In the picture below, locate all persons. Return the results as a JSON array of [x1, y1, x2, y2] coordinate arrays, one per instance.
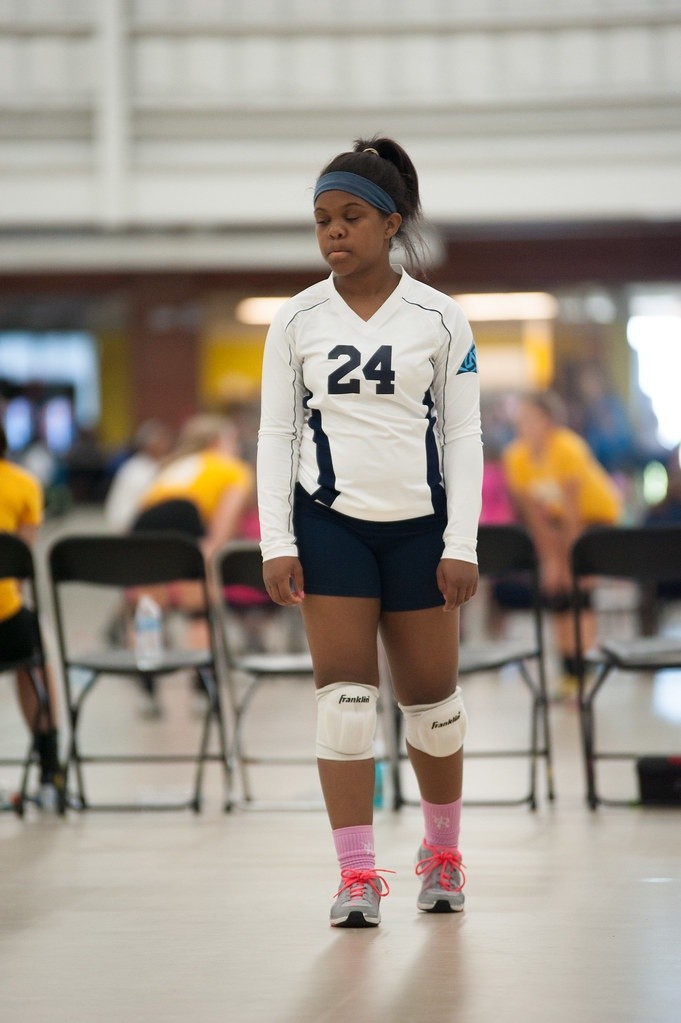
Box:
[[104, 350, 680, 718], [252, 135, 486, 924], [0, 422, 68, 790]]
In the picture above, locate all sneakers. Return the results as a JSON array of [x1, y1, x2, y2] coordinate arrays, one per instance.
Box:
[[330, 876, 383, 927], [418, 838, 466, 912]]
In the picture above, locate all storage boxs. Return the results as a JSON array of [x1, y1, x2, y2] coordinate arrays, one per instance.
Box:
[[636, 756, 681, 810]]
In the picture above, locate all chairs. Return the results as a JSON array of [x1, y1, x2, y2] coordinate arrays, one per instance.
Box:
[[388, 524, 558, 810], [48, 533, 237, 812], [217, 543, 319, 803], [562, 526, 681, 809], [0, 533, 71, 816]]
